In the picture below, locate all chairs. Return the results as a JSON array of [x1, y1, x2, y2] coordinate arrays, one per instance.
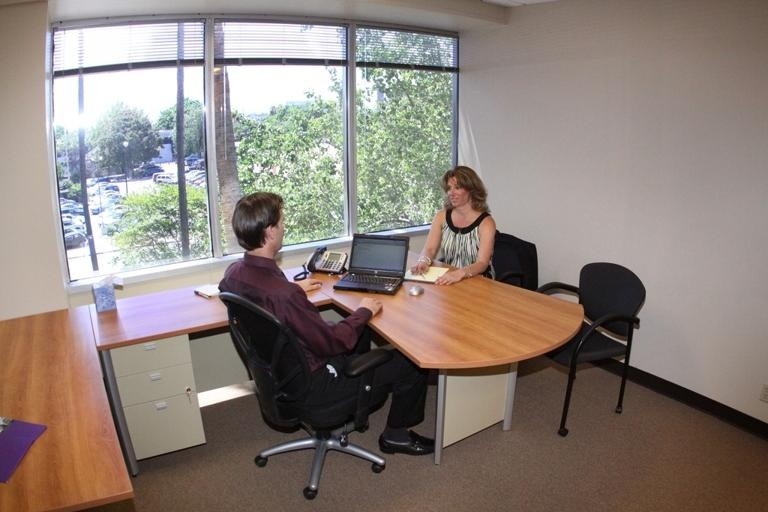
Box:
[[438, 226, 539, 287], [218, 293, 386, 501], [537, 261, 647, 437]]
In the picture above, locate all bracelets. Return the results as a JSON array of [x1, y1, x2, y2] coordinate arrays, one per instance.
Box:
[[417, 255, 432, 266], [463, 266, 472, 279]]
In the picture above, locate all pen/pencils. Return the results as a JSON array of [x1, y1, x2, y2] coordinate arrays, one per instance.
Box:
[[194, 290, 210, 299]]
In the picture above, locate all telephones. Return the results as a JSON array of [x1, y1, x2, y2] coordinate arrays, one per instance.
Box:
[[303, 246, 348, 274]]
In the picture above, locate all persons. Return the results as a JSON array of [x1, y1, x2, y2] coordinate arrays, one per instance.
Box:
[[218, 192, 437, 456], [411, 166, 497, 286]]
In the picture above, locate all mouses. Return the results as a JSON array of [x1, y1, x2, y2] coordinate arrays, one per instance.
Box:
[[408, 284, 424, 295]]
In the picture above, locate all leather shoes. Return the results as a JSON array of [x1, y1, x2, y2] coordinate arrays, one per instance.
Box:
[[379, 430, 434, 455]]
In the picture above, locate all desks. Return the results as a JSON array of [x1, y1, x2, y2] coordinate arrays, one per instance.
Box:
[[89, 272, 333, 476], [0, 305, 135, 512], [307, 247, 586, 465]]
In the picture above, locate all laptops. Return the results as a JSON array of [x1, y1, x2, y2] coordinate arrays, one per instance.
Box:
[[332, 232, 408, 298]]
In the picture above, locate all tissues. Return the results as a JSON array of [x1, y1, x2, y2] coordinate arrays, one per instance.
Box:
[[91, 273, 118, 314]]
